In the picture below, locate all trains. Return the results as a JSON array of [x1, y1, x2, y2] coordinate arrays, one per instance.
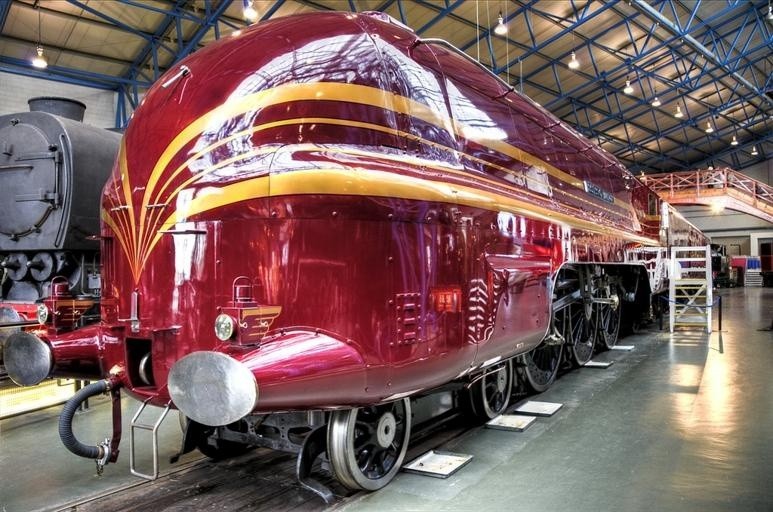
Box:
[[0, 90, 125, 353], [0, 2, 727, 510]]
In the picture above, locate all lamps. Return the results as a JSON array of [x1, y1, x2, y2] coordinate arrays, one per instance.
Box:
[[566, 14, 581, 70], [493, 0, 508, 36], [31, 0, 48, 68], [622, 67, 760, 157]]
